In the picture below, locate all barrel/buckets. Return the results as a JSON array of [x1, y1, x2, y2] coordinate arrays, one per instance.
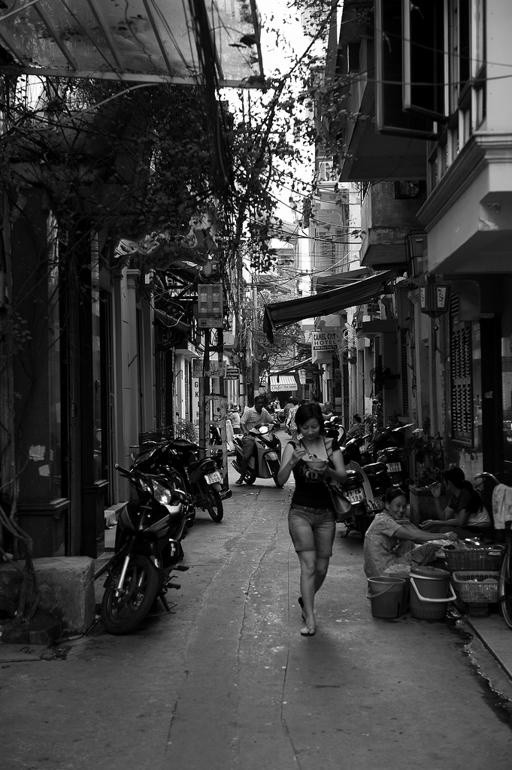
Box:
[[365, 574, 407, 619], [409, 566, 456, 619]]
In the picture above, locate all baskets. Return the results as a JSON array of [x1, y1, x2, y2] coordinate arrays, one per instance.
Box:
[[452, 571, 500, 602], [441, 545, 505, 570]]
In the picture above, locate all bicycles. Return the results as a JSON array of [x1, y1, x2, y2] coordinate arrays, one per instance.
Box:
[[474, 473, 511, 629]]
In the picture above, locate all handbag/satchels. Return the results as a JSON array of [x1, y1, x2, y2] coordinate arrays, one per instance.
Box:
[[323, 477, 351, 515]]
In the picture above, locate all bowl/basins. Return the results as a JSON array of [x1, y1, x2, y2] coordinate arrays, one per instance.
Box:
[[305, 460, 328, 470]]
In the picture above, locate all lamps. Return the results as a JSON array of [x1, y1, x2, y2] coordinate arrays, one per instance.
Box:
[[350, 335, 357, 365], [420, 270, 451, 359]]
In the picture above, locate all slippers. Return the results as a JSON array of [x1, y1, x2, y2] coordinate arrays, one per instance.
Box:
[[298, 597, 316, 636]]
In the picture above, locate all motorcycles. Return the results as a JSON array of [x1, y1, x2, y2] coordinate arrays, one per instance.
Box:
[[102, 437, 223, 634], [325, 415, 408, 537], [230, 420, 283, 488]]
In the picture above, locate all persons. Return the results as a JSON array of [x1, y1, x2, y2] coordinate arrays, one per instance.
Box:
[[419, 466, 491, 542], [277, 403, 346, 635], [226, 396, 365, 486], [364, 488, 458, 580]]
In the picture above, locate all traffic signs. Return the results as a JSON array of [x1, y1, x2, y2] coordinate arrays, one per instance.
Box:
[[221, 369, 240, 380], [312, 331, 340, 351]]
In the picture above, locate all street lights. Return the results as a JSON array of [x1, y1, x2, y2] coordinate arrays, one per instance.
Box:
[[419, 272, 449, 487], [200, 260, 220, 460]]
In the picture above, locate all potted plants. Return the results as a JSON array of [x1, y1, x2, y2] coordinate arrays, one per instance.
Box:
[[408, 454, 447, 529]]
